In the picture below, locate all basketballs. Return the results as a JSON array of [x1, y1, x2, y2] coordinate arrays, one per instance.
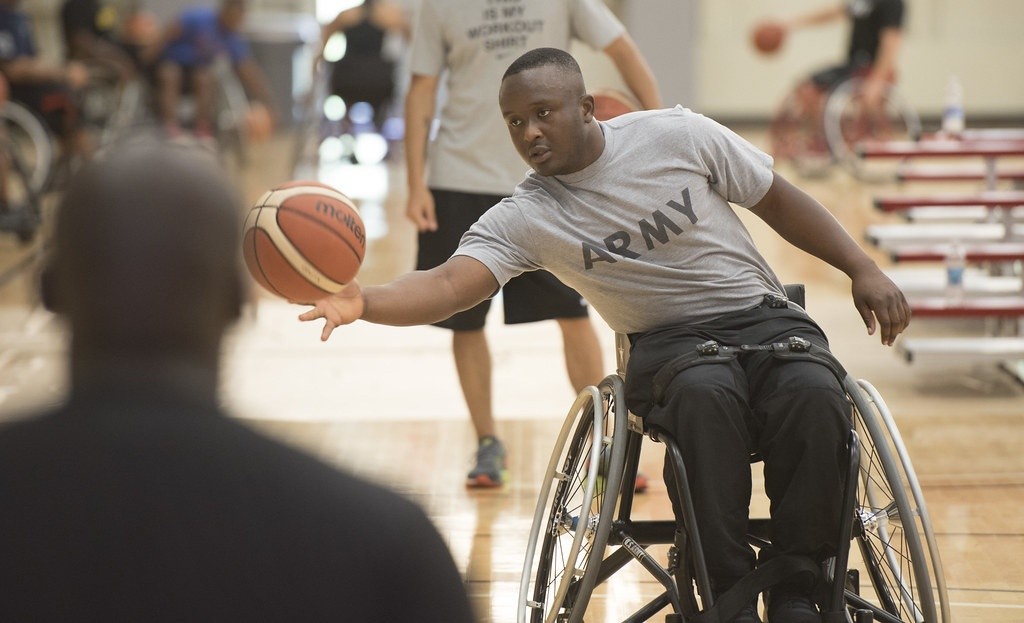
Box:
[[240, 179, 368, 303]]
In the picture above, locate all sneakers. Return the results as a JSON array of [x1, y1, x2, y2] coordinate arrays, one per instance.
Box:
[[465, 434, 509, 487], [585, 440, 648, 494]]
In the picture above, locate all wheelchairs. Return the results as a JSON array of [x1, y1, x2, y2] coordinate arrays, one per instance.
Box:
[[774, 66, 923, 181], [518, 284, 949, 623]]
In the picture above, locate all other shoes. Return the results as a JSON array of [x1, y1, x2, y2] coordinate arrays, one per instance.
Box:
[[768, 591, 822, 623], [725, 603, 762, 623]]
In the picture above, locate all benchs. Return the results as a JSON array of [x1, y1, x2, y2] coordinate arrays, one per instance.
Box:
[[858, 136, 1024, 386]]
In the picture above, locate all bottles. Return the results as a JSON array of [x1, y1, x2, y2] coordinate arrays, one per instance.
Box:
[[945, 244, 964, 299], [943, 82, 964, 137]]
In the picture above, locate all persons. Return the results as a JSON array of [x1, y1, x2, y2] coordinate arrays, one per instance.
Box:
[[0, 141, 475, 622], [287, 49, 910, 623], [312, 0, 405, 165], [406, 0, 665, 490], [0, 0, 269, 242], [775, 0, 905, 154]]
